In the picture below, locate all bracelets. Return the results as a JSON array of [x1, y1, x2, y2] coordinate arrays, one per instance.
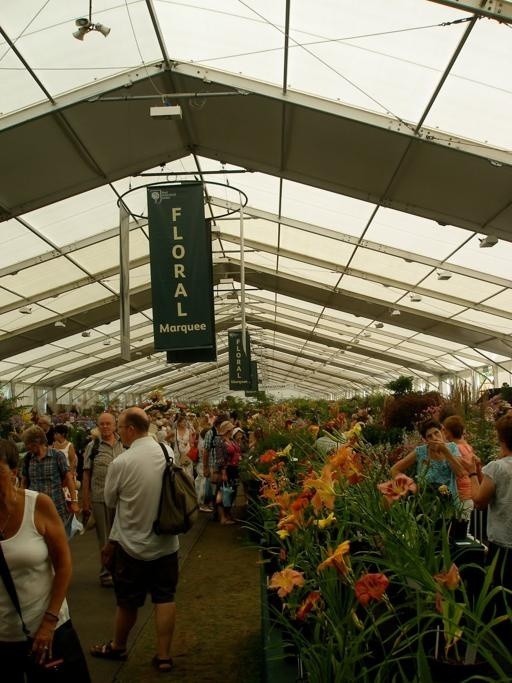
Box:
[[469, 472, 476, 477], [45, 610, 60, 623]]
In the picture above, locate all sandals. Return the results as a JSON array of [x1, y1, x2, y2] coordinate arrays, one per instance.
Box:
[[89, 639, 129, 662], [152, 653, 173, 673]]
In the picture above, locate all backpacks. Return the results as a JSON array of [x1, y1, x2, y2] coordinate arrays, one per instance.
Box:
[[78, 435, 99, 479], [152, 462, 200, 536]]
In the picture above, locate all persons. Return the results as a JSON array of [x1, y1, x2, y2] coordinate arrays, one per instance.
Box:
[[456, 410, 512, 585], [90, 407, 180, 671], [159, 400, 267, 528], [313, 407, 372, 454], [2, 438, 90, 682], [20, 418, 78, 545], [51, 424, 78, 479], [388, 417, 464, 547], [444, 414, 480, 522], [38, 416, 56, 445], [82, 412, 126, 586]]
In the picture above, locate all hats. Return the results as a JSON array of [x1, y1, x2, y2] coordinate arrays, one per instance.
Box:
[[65, 512, 85, 542], [231, 427, 246, 440], [219, 421, 237, 435]]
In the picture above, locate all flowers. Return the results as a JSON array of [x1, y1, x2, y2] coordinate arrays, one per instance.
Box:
[[1, 389, 236, 453]]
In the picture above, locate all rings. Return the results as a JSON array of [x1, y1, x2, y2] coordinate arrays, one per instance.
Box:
[[45, 643, 51, 650]]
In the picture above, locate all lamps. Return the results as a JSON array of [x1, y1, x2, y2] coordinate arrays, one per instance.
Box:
[[17, 304, 214, 384], [72, 0, 110, 42], [292, 233, 498, 389]]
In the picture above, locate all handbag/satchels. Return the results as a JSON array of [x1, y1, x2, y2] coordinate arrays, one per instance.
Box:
[[220, 487, 235, 507], [178, 452, 191, 466], [421, 482, 451, 510], [24, 642, 65, 683], [187, 446, 199, 463]]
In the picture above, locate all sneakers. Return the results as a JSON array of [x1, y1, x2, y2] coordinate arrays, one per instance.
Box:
[[201, 506, 213, 512], [99, 566, 114, 586]]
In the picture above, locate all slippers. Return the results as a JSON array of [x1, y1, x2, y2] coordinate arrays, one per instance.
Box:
[[221, 519, 236, 525]]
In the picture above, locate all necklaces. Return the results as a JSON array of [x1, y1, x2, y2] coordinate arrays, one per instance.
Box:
[[1, 489, 20, 536], [55, 439, 67, 445]]
